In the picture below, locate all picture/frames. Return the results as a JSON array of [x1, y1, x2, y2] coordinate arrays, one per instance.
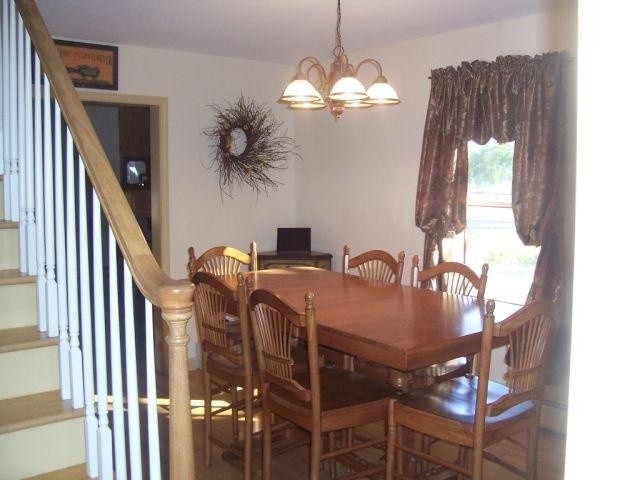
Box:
[[31, 37, 119, 92]]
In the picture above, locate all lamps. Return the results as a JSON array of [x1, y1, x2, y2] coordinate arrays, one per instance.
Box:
[[279, 0, 401, 122]]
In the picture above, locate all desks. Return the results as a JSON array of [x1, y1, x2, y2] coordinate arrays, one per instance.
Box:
[[214, 266, 526, 480]]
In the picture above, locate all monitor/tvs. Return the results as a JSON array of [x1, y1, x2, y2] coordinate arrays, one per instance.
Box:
[[123, 156, 151, 189]]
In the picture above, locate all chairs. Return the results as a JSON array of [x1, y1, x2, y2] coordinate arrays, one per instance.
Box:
[[186, 242, 258, 348], [298, 244, 405, 449], [244, 276, 410, 480], [392, 286, 562, 479], [189, 261, 290, 480], [352, 254, 488, 468]]
